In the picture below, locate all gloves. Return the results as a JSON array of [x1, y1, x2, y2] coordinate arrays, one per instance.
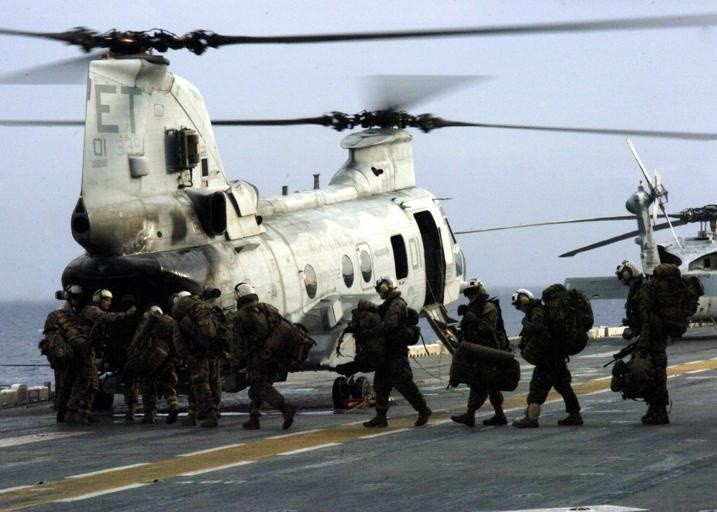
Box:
[[458, 305, 468, 315]]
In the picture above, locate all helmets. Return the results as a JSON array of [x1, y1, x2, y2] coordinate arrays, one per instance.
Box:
[[616, 261, 641, 286], [512, 289, 535, 311], [64, 283, 258, 318], [375, 276, 397, 300], [464, 279, 484, 297]]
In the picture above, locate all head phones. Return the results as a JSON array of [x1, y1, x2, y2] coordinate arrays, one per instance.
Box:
[[172, 293, 179, 306], [64, 284, 72, 299], [622, 259, 630, 280], [472, 278, 486, 295], [234, 282, 255, 301], [513, 289, 529, 305], [376, 276, 389, 292], [93, 289, 103, 303]]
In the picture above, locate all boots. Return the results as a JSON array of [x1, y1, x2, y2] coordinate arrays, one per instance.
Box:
[[363, 404, 389, 427], [483, 415, 507, 426], [642, 405, 669, 425], [282, 404, 296, 429], [512, 414, 539, 428], [242, 410, 262, 429], [415, 406, 432, 426], [57, 404, 221, 427], [558, 412, 583, 426], [451, 414, 475, 427]]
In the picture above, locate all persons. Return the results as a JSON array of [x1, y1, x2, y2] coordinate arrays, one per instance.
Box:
[[511, 289, 584, 428], [451, 278, 508, 426], [615, 261, 670, 423], [359, 277, 432, 428], [42, 281, 297, 430]]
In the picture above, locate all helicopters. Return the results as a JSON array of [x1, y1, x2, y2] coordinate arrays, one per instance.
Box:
[[0, 11, 716, 412], [556, 135, 716, 338]]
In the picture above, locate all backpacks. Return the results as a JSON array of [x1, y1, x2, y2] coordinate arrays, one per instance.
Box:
[[653, 263, 704, 338], [407, 308, 419, 345], [542, 284, 593, 355]]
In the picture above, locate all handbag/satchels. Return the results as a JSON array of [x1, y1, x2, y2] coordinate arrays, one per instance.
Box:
[[134, 334, 169, 371], [278, 319, 315, 362]]
